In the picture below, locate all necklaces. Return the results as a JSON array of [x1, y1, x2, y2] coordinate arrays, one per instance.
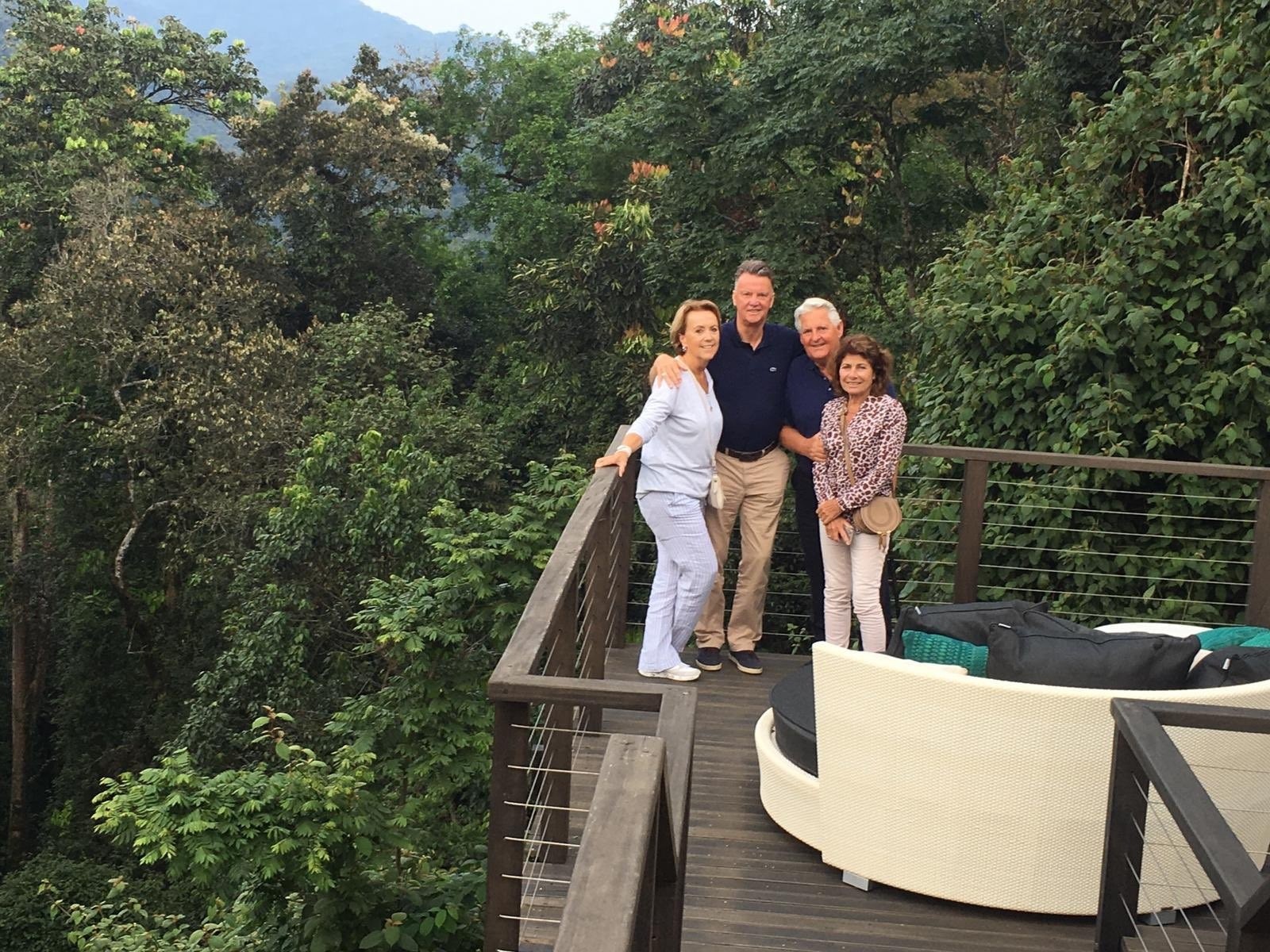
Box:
[[847, 411, 856, 415]]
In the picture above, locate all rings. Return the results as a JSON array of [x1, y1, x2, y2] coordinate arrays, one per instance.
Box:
[[661, 372, 667, 376], [827, 535, 831, 538]]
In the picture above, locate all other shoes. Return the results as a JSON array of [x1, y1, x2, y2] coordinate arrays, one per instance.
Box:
[[728, 650, 763, 674], [695, 647, 722, 671], [638, 662, 701, 681]]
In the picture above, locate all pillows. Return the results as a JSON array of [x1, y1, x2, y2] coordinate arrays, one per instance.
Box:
[[888, 600, 1048, 658], [901, 629, 988, 675], [985, 610, 1270, 690]]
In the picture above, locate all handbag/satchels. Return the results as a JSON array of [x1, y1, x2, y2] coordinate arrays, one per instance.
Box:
[[853, 495, 902, 554], [708, 474, 726, 509]]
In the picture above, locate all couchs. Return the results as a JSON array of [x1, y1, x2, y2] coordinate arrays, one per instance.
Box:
[[753, 622, 1269, 926]]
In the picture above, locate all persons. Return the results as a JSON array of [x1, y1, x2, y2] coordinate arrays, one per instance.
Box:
[[595, 299, 722, 681], [780, 298, 898, 651], [811, 333, 907, 655], [650, 259, 806, 674]]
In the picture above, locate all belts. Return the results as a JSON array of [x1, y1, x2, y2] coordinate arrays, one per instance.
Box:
[[716, 438, 779, 462]]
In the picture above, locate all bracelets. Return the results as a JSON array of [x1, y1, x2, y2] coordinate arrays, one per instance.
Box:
[[616, 445, 632, 457]]
